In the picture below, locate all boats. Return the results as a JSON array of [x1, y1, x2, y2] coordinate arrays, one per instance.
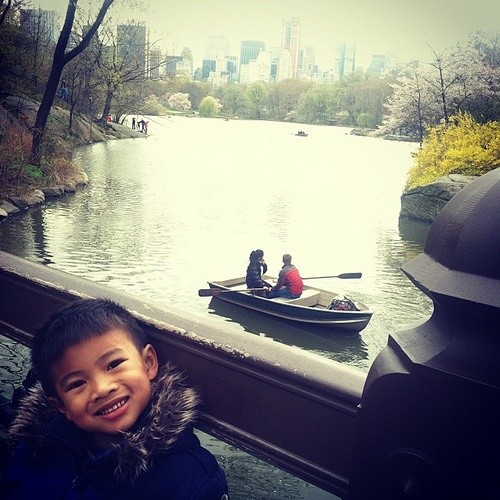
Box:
[[294, 131, 308, 137], [208, 273, 373, 331]]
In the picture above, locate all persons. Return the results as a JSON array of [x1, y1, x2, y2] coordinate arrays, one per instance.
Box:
[[267, 255, 304, 299], [0, 298, 230, 500], [108, 115, 111, 122], [246, 249, 272, 297], [132, 118, 148, 133]]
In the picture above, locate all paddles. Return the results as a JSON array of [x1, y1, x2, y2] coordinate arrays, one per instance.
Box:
[[302, 273, 361, 278], [199, 288, 266, 297]]
